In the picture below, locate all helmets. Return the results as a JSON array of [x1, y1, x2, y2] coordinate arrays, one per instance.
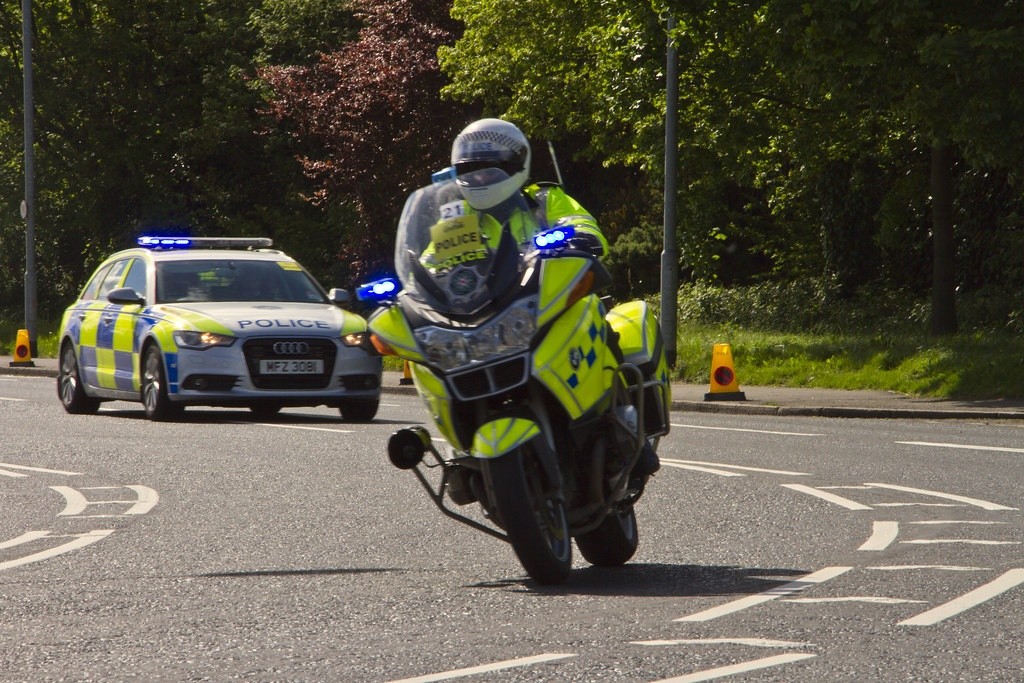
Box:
[[451, 118, 531, 210]]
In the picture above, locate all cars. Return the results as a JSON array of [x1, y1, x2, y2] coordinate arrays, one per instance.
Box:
[[54, 237, 384, 422]]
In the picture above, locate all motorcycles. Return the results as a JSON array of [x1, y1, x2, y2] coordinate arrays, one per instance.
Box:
[[355, 166, 673, 584]]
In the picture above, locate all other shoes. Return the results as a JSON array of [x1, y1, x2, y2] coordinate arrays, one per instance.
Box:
[[630, 437, 660, 478]]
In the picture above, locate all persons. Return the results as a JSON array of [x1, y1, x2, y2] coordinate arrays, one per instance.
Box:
[[402, 118, 660, 506]]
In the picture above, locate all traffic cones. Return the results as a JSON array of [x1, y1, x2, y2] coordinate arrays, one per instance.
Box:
[[702, 343, 749, 403], [398, 356, 415, 385], [8, 329, 36, 368]]
